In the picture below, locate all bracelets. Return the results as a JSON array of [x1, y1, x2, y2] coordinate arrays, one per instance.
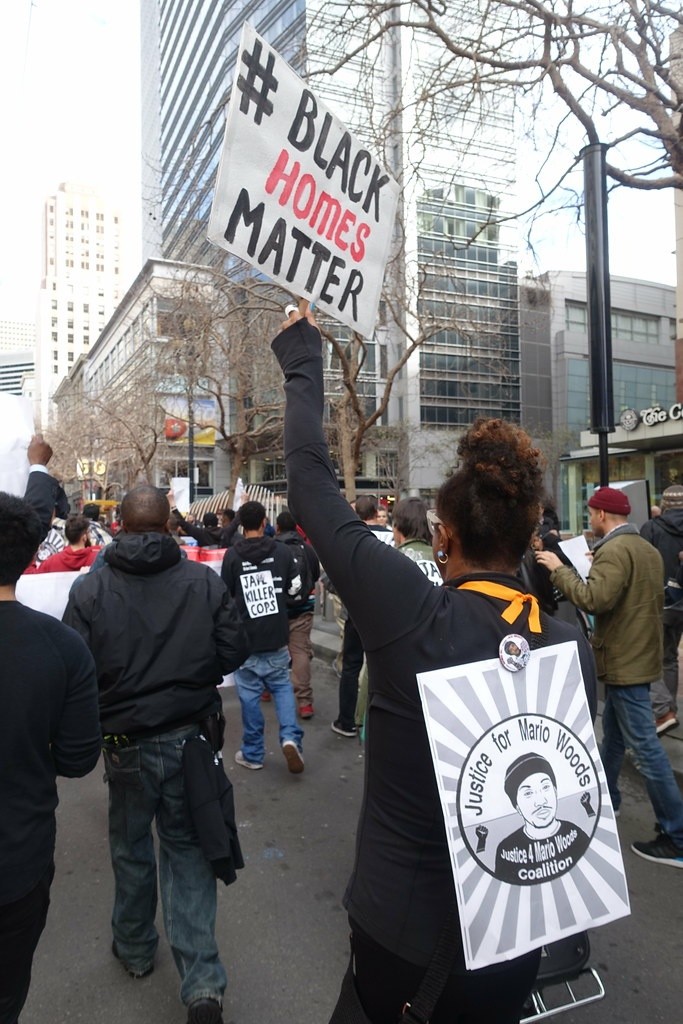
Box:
[[171, 506, 176, 509]]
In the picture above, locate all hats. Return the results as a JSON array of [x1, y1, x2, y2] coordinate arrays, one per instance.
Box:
[[660, 485, 683, 509], [589, 487, 631, 515]]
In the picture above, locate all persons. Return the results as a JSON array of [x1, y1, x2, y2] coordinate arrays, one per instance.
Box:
[[63, 487, 253, 1023], [165, 490, 444, 737], [519, 497, 588, 638], [0, 491, 102, 1024], [220, 501, 306, 774], [0, 435, 124, 575], [640, 486, 683, 735], [534, 486, 683, 868], [272, 304, 597, 1023], [504, 641, 521, 657]]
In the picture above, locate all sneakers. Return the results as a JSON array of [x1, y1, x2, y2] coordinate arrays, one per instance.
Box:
[[631, 829, 683, 868], [235, 751, 264, 770], [283, 741, 305, 773], [614, 807, 621, 817]]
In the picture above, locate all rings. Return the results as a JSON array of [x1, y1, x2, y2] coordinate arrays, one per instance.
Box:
[[284, 305, 299, 318]]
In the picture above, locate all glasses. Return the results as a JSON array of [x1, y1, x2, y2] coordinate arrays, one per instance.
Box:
[[426, 509, 444, 536]]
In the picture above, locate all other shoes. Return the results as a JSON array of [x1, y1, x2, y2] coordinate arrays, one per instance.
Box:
[[189, 997, 224, 1024], [331, 720, 356, 736], [112, 940, 156, 977], [261, 690, 271, 701], [656, 711, 679, 737], [301, 704, 314, 717]]
